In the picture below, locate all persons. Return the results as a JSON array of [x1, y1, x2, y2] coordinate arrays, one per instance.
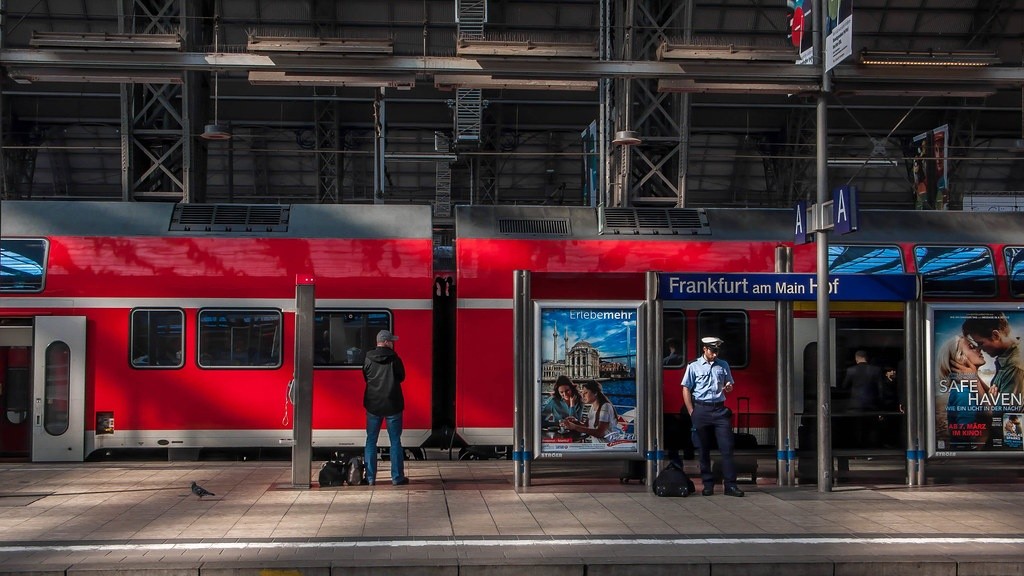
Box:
[[845, 351, 906, 460], [681, 336, 744, 496], [933, 132, 945, 210], [542, 376, 618, 442], [939, 313, 1024, 448], [910, 140, 929, 210], [664, 337, 683, 366], [362, 330, 409, 485]]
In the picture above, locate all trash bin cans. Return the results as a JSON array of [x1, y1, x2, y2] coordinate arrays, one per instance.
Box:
[[916, 189, 948, 211]]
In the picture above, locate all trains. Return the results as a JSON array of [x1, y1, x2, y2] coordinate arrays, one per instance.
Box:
[[0, 198, 1024, 465]]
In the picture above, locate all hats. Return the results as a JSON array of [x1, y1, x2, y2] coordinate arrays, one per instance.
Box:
[[666, 337, 681, 342], [376, 330, 398, 342], [701, 336, 724, 352]]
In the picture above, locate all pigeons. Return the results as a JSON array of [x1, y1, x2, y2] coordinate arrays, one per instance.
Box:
[[190, 481, 216, 500]]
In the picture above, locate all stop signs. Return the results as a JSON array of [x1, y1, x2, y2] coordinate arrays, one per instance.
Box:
[[792, 7, 805, 49]]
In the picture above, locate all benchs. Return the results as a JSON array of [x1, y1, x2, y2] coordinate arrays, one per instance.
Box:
[[833, 449, 906, 459], [664, 449, 778, 463]]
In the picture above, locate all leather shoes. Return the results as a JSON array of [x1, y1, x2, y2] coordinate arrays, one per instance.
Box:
[[725, 486, 744, 496], [702, 487, 714, 496]]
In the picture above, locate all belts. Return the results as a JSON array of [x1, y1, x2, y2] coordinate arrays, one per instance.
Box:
[[694, 400, 724, 407]]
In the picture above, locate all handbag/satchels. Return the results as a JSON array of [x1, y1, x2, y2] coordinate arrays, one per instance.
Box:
[[653, 462, 695, 497], [319, 457, 348, 487]]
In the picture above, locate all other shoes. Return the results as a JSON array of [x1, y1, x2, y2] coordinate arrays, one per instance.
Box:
[[392, 478, 409, 485], [369, 481, 375, 485]]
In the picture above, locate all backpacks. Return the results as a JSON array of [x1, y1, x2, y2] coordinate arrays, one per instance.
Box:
[[346, 456, 366, 485]]
